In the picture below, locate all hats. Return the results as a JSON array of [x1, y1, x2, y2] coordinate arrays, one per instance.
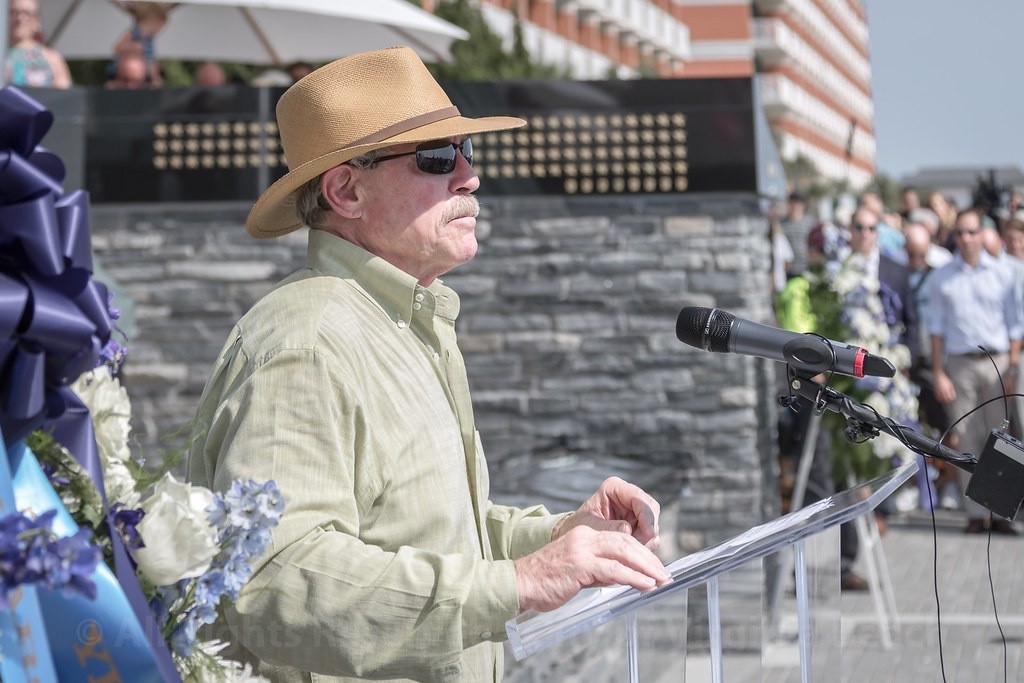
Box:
[[242, 48, 524, 242], [902, 205, 940, 236], [809, 222, 840, 250]]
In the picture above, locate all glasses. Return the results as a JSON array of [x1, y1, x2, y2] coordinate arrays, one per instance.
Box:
[[352, 137, 477, 174], [954, 226, 979, 239], [852, 222, 877, 232]]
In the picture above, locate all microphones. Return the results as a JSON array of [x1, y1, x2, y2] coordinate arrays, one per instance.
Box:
[[676, 306, 896, 380]]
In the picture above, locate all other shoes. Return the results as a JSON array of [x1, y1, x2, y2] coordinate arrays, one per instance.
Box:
[[966, 514, 1017, 538], [939, 479, 960, 511], [839, 574, 872, 591]]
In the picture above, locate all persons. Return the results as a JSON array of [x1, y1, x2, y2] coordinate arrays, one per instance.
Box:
[[102, 4, 314, 88], [2, 1, 71, 88], [189, 48, 672, 681], [775, 187, 1024, 591]]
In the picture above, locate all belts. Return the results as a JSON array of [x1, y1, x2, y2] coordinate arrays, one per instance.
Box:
[[960, 349, 998, 360]]
